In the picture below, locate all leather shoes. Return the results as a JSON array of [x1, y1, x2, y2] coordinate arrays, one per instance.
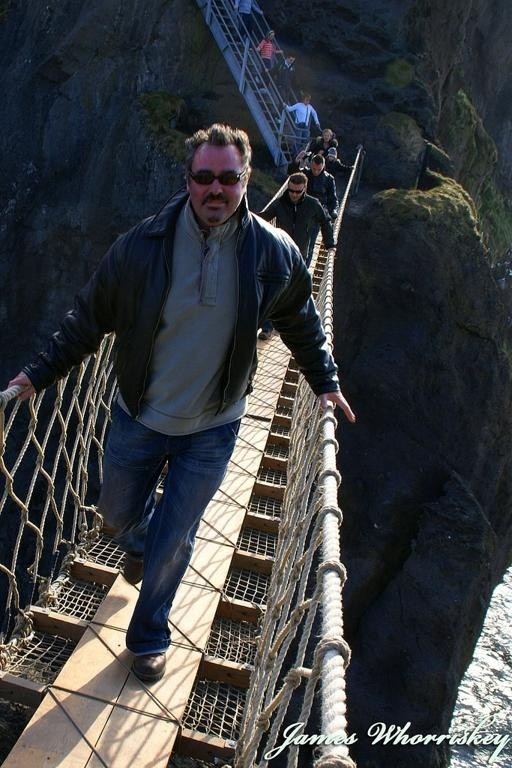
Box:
[[123, 556, 146, 584], [133, 651, 167, 681]]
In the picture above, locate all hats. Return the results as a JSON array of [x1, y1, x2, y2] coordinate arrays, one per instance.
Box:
[[327, 146, 337, 159]]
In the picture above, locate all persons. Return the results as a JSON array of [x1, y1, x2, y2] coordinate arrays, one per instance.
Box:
[[264, 52, 296, 94], [322, 145, 356, 175], [232, 0, 264, 42], [286, 149, 338, 270], [282, 92, 323, 152], [252, 173, 338, 342], [6, 120, 357, 684], [306, 127, 339, 154], [255, 29, 284, 91]]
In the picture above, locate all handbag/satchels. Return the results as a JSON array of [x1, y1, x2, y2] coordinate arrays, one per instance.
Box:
[[298, 121, 306, 127]]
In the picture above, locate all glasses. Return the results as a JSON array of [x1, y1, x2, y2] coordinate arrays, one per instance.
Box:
[[188, 166, 248, 186], [287, 186, 305, 194]]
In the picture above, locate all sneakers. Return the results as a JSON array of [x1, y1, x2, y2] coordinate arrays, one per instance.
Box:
[[259, 330, 271, 340]]
